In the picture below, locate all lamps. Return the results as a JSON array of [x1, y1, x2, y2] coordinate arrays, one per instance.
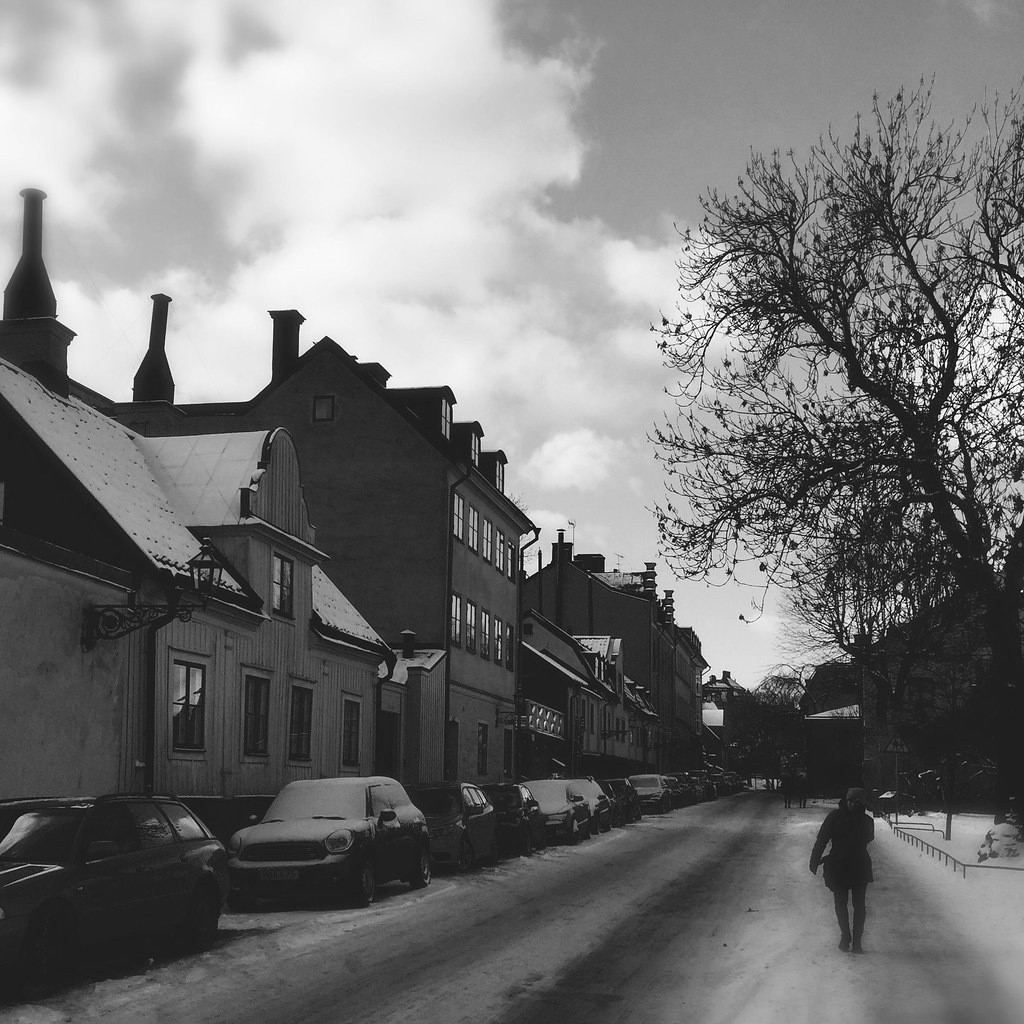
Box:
[[496, 684, 526, 728], [81, 537, 223, 654], [601, 713, 637, 740]]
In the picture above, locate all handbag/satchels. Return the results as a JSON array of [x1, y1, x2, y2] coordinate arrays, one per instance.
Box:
[[833, 836, 870, 872]]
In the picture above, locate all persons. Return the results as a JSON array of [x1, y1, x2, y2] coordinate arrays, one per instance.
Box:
[[809, 786, 874, 953], [782, 766, 808, 809]]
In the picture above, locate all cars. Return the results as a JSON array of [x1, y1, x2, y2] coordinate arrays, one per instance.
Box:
[[402, 780, 495, 875], [0, 791, 230, 996], [478, 783, 547, 858], [517, 766, 748, 845], [227, 776, 433, 908]]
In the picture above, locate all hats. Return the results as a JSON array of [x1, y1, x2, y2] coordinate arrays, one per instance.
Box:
[[845, 788, 865, 802]]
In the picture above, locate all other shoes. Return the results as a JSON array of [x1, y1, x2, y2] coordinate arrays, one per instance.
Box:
[[839, 933, 852, 952], [849, 946, 863, 954]]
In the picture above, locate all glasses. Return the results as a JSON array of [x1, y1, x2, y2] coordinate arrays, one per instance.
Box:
[[847, 799, 860, 808]]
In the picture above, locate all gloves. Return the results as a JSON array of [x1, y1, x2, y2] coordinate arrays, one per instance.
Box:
[[809, 862, 818, 875]]
